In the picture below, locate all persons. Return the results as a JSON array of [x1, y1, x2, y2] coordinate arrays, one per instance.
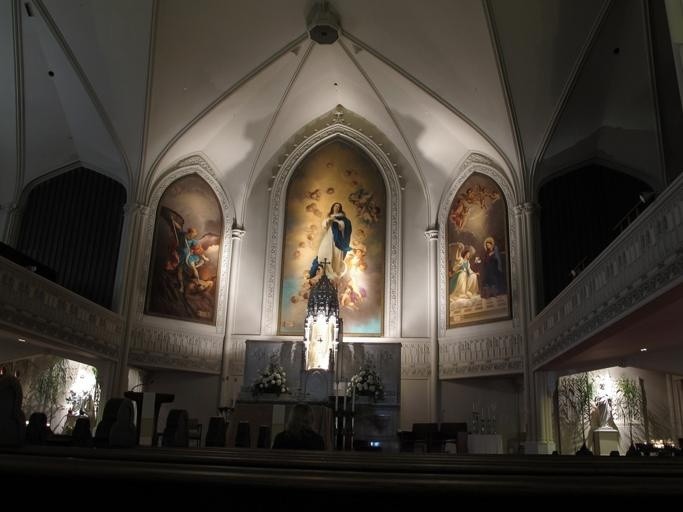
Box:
[[480, 235, 503, 302], [163, 214, 210, 295], [307, 200, 352, 289], [452, 245, 483, 302], [270, 400, 327, 451]]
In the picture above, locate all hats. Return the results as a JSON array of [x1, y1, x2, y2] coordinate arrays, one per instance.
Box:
[[486, 240, 492, 244], [461, 250, 468, 257]]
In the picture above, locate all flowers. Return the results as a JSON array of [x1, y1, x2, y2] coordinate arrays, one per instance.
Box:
[[251, 361, 290, 397], [346, 366, 387, 403]]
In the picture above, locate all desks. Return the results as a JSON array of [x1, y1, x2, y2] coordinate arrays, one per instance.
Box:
[[469, 434, 503, 455]]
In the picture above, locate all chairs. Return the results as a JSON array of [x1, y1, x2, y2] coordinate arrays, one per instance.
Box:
[[188, 424, 202, 448]]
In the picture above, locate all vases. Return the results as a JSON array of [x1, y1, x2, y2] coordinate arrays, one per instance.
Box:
[[355, 395, 374, 404], [255, 392, 276, 402]]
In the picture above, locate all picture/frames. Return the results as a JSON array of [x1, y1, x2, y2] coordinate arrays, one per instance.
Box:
[[144, 172, 224, 327], [276, 135, 384, 337], [446, 171, 512, 329]]
